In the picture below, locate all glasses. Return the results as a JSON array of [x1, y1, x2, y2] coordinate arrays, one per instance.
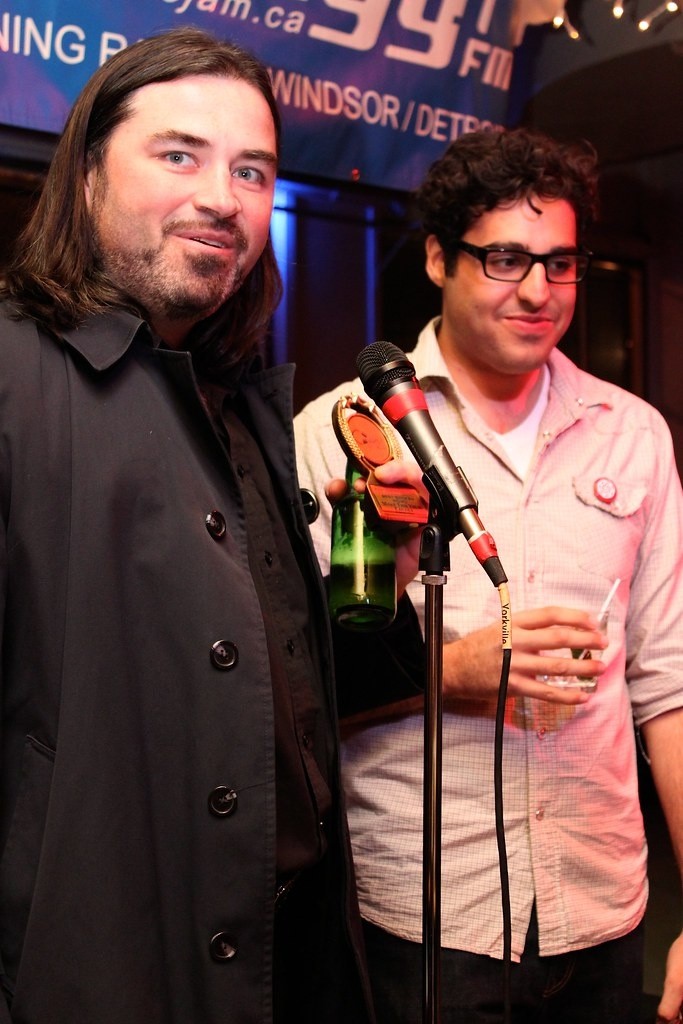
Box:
[[457, 235, 593, 285]]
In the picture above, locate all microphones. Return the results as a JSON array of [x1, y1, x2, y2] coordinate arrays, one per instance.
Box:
[[359, 338, 511, 587]]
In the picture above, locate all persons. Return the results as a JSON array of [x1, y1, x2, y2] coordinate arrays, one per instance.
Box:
[[0, 31, 360, 1021], [293, 125, 683, 1022]]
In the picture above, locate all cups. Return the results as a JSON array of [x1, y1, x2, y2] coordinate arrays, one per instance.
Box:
[[535, 604, 609, 686]]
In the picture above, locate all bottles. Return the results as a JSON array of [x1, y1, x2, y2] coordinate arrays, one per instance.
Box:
[[331, 457, 396, 632]]
